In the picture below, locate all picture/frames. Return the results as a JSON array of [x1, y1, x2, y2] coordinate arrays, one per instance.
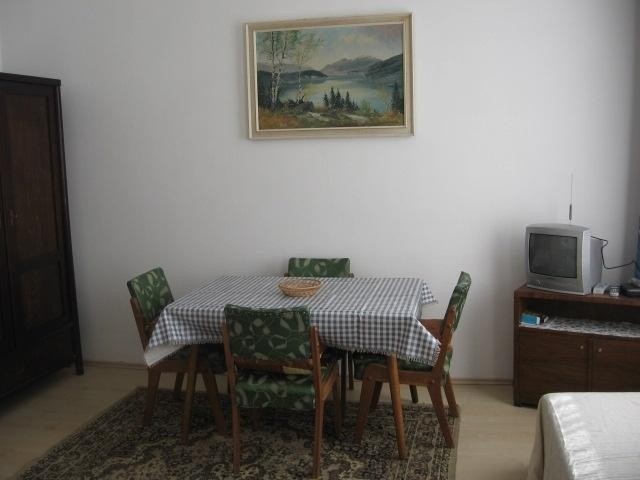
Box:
[[245, 13, 413, 140]]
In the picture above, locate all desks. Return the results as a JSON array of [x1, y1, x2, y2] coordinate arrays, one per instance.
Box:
[[162, 274, 424, 460]]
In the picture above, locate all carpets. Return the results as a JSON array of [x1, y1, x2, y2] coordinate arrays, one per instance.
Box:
[[7, 384, 461, 480]]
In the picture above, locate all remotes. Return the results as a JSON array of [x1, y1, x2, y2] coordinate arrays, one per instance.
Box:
[[593, 282, 609, 295], [610, 285, 620, 297]]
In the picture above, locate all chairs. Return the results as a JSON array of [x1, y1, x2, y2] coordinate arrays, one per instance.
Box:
[[284, 258, 355, 404], [350, 271, 471, 448], [219, 303, 343, 477], [127, 267, 229, 435]]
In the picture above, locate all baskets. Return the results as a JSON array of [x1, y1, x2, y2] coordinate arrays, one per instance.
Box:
[[278, 279, 323, 297]]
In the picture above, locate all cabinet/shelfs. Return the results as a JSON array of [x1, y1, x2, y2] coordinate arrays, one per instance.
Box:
[[1, 74, 85, 400], [514, 301, 639, 408]]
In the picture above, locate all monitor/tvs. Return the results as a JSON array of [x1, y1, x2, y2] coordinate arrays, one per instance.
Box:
[[524, 223, 603, 295]]
